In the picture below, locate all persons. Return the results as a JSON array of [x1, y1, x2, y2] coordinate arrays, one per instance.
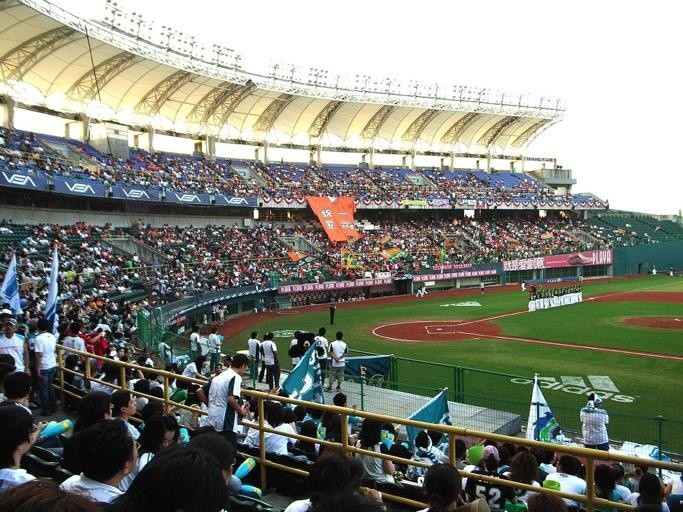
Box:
[[211, 302, 227, 326], [447, 440, 466, 469], [16, 323, 29, 337], [176, 356, 206, 390], [481, 211, 660, 262], [0, 354, 17, 403], [210, 364, 223, 377], [0, 318, 32, 376], [110, 443, 231, 512], [416, 281, 428, 299], [0, 372, 32, 415], [169, 390, 188, 420], [89, 329, 108, 373], [1, 478, 104, 511], [197, 354, 249, 473], [329, 303, 336, 325], [119, 416, 178, 492], [314, 328, 329, 387], [414, 463, 467, 512], [426, 430, 450, 462], [579, 272, 582, 281], [480, 280, 485, 294], [63, 355, 91, 391], [188, 433, 242, 493], [16, 246, 48, 318], [77, 391, 112, 421], [520, 278, 526, 291], [190, 326, 202, 356], [247, 332, 260, 380], [66, 319, 106, 344], [139, 402, 164, 430], [59, 419, 139, 503], [407, 432, 444, 483], [652, 266, 657, 276], [468, 439, 682, 511], [378, 422, 412, 476], [186, 382, 209, 427], [191, 425, 214, 435], [355, 418, 406, 494], [413, 210, 480, 274], [528, 284, 583, 312], [670, 265, 674, 277], [57, 221, 135, 269], [93, 319, 112, 334], [57, 266, 162, 318], [135, 214, 413, 295], [34, 319, 58, 416], [306, 491, 384, 511], [223, 355, 232, 368], [112, 390, 141, 440], [109, 318, 176, 397], [0, 404, 37, 494], [239, 389, 360, 465], [324, 332, 348, 392], [580, 393, 610, 450], [202, 312, 208, 334], [61, 322, 87, 372], [290, 331, 301, 367], [263, 333, 281, 390], [0, 314, 14, 336], [1, 219, 58, 246], [281, 451, 388, 512], [26, 319, 39, 408], [258, 335, 270, 384], [208, 327, 223, 371]]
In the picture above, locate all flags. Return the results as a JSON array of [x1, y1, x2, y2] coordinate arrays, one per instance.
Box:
[[326, 354, 392, 388], [281, 341, 324, 403], [1, 252, 23, 315], [44, 244, 59, 335], [406, 391, 455, 456], [526, 377, 577, 449]]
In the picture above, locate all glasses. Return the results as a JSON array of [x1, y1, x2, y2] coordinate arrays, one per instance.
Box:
[[136, 441, 141, 450], [232, 457, 237, 467], [163, 439, 176, 443], [29, 427, 36, 433], [109, 403, 116, 409]]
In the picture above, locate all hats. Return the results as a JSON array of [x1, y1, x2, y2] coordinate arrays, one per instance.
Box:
[[1, 308, 12, 316], [482, 445, 501, 462], [18, 323, 29, 329], [6, 318, 18, 326]]
